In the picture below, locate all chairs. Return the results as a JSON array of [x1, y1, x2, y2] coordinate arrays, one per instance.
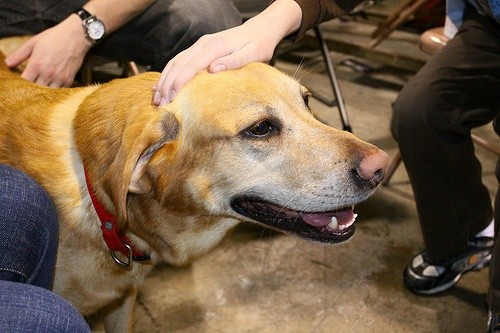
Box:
[[75, 51, 140, 87], [227, 0, 354, 138], [377, 19, 500, 186]]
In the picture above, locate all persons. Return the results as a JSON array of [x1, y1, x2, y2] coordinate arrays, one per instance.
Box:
[[0, 0, 244, 93], [153, 1, 499, 296], [0, 162, 93, 333]]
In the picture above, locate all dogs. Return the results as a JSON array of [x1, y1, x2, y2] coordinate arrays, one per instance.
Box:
[[0, 48, 391, 333]]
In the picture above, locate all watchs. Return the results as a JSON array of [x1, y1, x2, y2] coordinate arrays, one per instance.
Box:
[[75, 6, 107, 45]]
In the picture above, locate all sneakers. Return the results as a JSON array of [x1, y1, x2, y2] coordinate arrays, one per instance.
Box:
[[486, 287, 500, 332], [404, 236, 495, 294]]
